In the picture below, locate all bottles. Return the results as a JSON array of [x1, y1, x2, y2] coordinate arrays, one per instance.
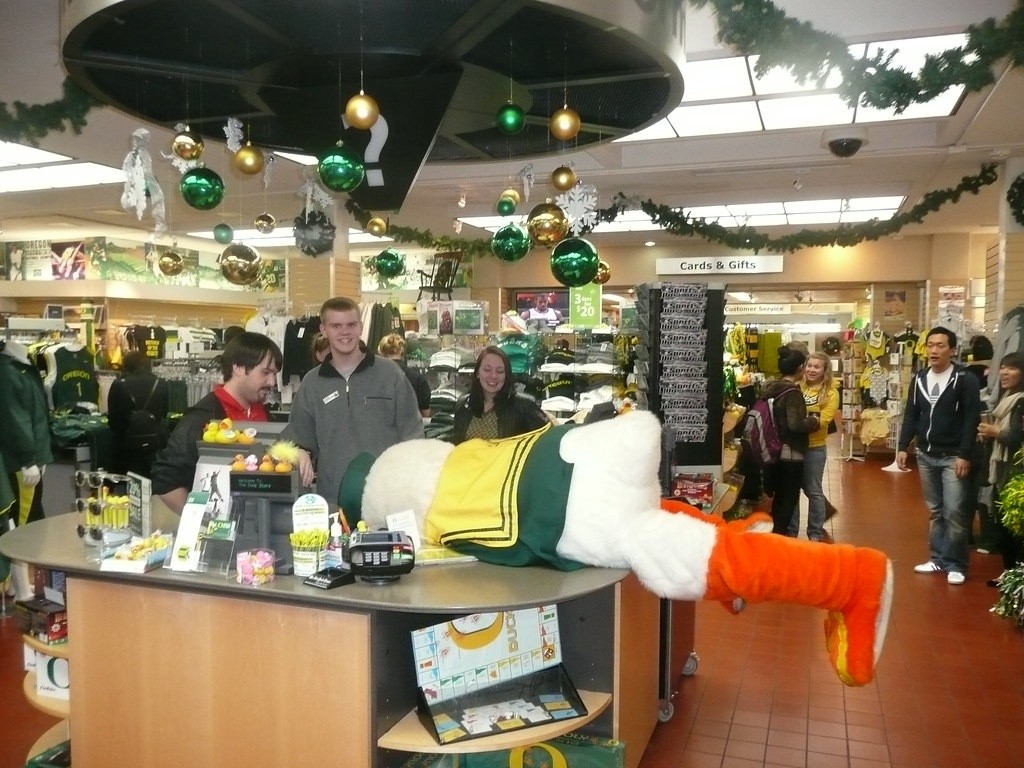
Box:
[[980, 410, 993, 426]]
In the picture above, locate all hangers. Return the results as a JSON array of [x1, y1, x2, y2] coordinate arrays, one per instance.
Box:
[[257, 296, 320, 319], [932, 315, 973, 344], [360, 289, 392, 306], [868, 320, 913, 409], [0, 314, 224, 386]]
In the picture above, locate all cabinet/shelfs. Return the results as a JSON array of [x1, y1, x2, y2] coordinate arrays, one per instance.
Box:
[[881, 341, 915, 473], [405, 333, 743, 519], [833, 340, 867, 461]]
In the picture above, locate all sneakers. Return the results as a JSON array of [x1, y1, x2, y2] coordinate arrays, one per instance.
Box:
[[947, 571, 965, 584], [914, 562, 943, 573]]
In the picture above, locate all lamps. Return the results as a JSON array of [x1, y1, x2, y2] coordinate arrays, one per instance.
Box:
[[458, 192, 466, 208], [794, 293, 803, 301], [793, 180, 805, 192], [809, 297, 814, 308], [866, 288, 871, 299]]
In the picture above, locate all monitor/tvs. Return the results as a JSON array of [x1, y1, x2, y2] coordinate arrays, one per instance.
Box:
[[197, 440, 305, 575], [511, 288, 571, 330]]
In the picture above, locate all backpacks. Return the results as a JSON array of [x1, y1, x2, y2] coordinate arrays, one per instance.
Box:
[[741, 388, 803, 466], [120, 378, 159, 452]]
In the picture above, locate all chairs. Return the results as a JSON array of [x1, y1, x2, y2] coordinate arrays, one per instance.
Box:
[[413, 252, 463, 310]]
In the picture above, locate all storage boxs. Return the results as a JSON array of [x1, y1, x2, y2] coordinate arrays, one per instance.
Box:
[[16, 599, 69, 647], [37, 651, 69, 700], [672, 473, 713, 507], [23, 642, 37, 671], [466, 735, 625, 768], [400, 753, 466, 768]]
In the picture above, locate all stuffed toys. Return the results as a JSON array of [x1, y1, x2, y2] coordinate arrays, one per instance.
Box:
[[336, 405, 893, 688]]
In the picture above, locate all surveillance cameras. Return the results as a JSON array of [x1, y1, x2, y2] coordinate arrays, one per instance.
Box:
[[820, 126, 870, 157]]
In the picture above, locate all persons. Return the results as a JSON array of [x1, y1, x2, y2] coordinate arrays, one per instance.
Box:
[[277, 297, 426, 521], [312, 333, 330, 366], [760, 340, 840, 545], [200, 472, 211, 492], [976, 352, 1024, 584], [107, 350, 168, 476], [377, 334, 431, 416], [0, 341, 54, 602], [90, 240, 106, 276], [453, 346, 554, 446], [51, 241, 85, 280], [149, 329, 315, 518], [440, 308, 452, 333], [145, 244, 166, 282], [10, 245, 23, 280], [896, 326, 980, 585], [520, 293, 563, 320], [209, 470, 224, 501]]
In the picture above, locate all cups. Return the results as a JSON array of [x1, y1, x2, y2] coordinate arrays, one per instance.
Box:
[[236, 548, 275, 585], [809, 412, 820, 428], [289, 539, 331, 577]]
